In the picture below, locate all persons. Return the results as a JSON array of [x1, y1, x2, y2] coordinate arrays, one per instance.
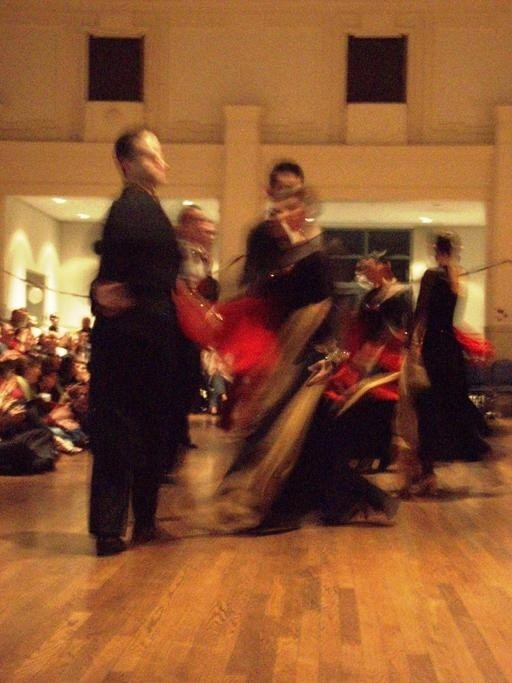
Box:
[[2, 308, 91, 454], [407, 236, 494, 463], [87, 129, 438, 557]]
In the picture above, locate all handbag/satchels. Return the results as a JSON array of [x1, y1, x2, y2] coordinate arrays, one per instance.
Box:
[[0, 412, 55, 474]]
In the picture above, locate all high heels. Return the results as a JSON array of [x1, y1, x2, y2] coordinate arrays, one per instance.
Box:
[[318, 493, 370, 523], [409, 475, 437, 494]]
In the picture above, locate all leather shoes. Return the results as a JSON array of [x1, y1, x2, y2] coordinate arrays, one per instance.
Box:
[[96, 534, 128, 555], [132, 520, 180, 544]]
